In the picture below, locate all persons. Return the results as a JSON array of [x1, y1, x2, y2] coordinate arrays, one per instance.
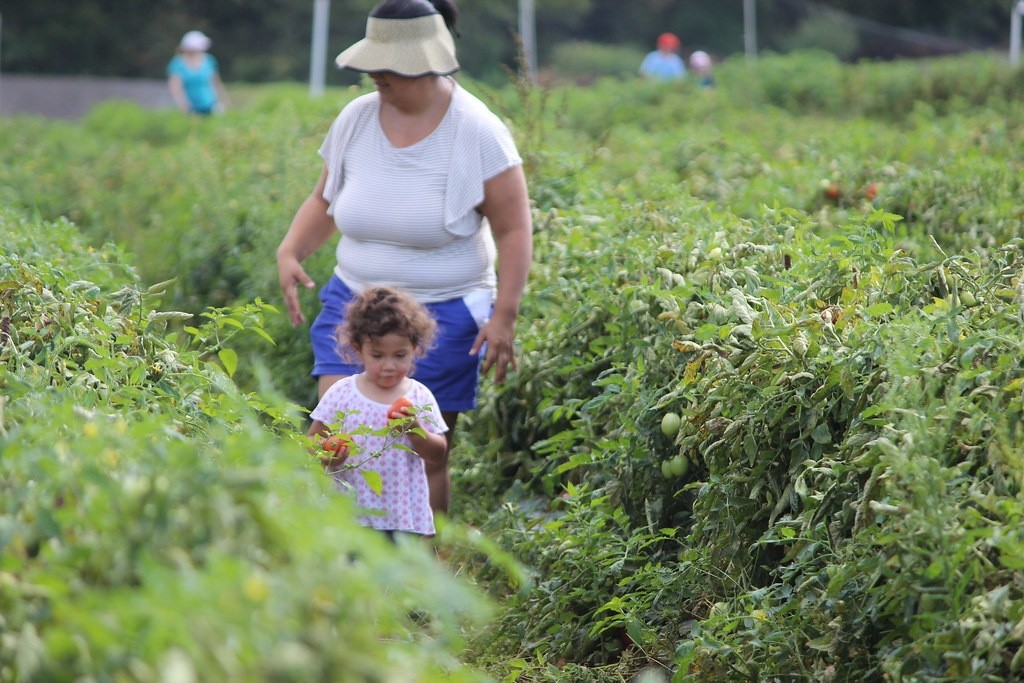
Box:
[[304, 285, 450, 566], [689, 49, 714, 88], [167, 29, 231, 118], [277, 0, 537, 561], [636, 32, 688, 86]]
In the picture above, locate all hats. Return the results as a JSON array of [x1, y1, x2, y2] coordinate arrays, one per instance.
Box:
[[334, 13, 460, 77], [179, 30, 213, 51]]
